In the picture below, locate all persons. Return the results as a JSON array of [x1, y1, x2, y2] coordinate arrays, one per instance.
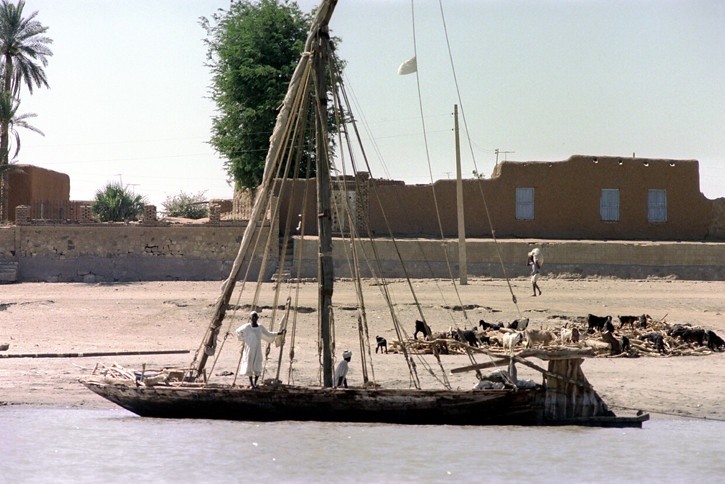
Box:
[[527, 256, 542, 296], [236, 311, 286, 387], [334, 351, 352, 387]]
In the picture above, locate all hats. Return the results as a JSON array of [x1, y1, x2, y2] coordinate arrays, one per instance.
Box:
[[343, 351, 352, 358]]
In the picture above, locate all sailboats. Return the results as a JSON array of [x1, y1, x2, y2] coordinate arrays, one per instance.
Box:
[[75, 0, 652, 429]]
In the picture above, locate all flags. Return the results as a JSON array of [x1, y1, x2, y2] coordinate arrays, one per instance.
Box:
[[398, 57, 417, 75]]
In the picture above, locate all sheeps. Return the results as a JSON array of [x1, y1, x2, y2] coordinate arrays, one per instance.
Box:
[[413, 320, 433, 341], [375, 334, 389, 355], [448, 311, 725, 357]]
